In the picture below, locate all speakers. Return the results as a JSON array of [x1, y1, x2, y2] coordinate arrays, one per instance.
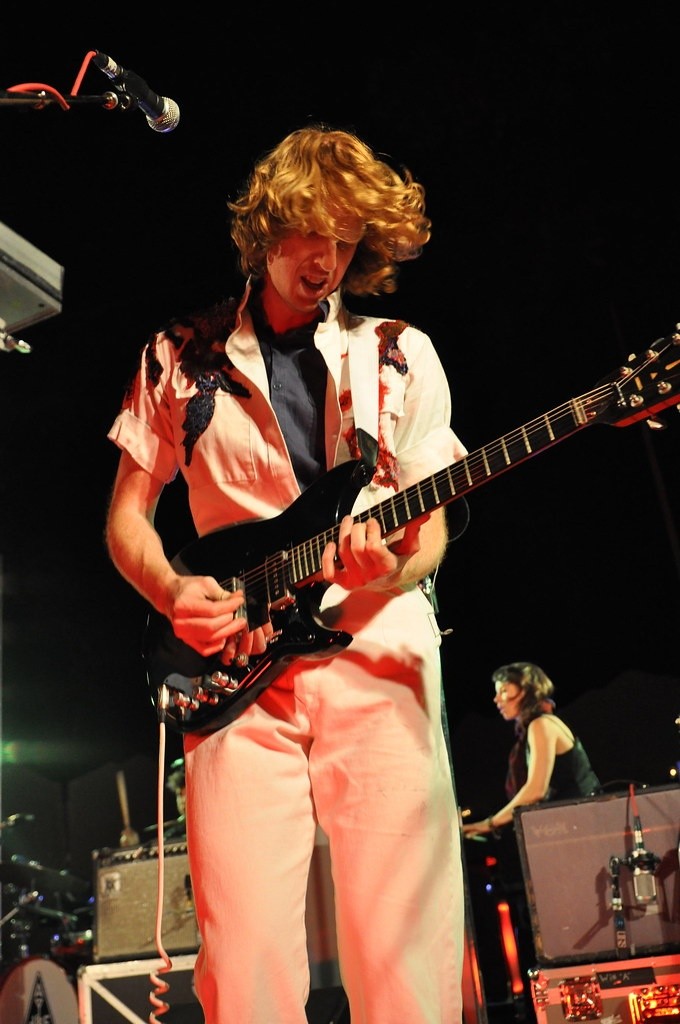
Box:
[[92, 840, 203, 964], [513, 781, 680, 969]]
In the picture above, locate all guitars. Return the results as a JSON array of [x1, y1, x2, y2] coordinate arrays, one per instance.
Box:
[[138, 322, 680, 733]]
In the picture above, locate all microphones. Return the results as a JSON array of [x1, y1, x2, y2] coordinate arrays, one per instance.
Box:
[[183, 874, 194, 902], [632, 815, 657, 905], [92, 50, 180, 132]]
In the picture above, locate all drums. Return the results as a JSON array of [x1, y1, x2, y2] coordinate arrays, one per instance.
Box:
[[0, 953, 79, 1024]]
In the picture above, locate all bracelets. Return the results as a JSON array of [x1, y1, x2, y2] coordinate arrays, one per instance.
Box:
[[486, 814, 496, 829]]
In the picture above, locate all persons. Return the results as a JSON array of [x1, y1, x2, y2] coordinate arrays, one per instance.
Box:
[[116, 755, 186, 845], [463, 660, 604, 834], [111, 130, 471, 1024]]
